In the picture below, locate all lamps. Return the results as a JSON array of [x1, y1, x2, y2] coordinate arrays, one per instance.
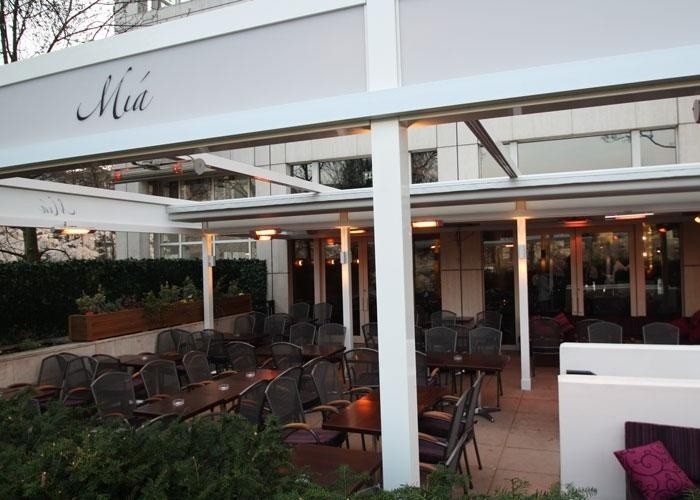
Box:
[[51, 228, 97, 235]]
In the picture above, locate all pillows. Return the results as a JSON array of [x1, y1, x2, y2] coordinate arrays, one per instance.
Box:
[[613, 439, 697, 499]]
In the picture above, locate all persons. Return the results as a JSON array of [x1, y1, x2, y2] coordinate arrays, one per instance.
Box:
[[415, 268, 441, 324], [612, 256, 628, 282], [652, 250, 661, 265]]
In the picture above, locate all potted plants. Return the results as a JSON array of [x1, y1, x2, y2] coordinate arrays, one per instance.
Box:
[[68, 276, 252, 344]]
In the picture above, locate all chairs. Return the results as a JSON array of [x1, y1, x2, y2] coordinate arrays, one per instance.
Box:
[[624, 421, 700, 500], [529, 312, 681, 376]]
